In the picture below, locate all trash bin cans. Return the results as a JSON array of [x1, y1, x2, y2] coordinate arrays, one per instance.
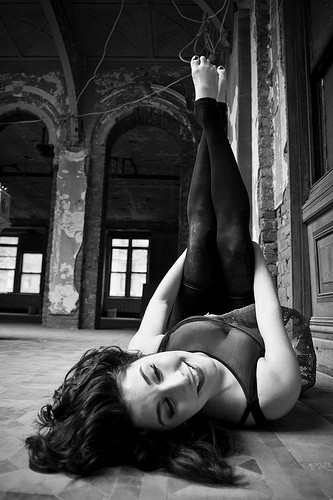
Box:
[[27, 303, 37, 315], [107, 306, 117, 317]]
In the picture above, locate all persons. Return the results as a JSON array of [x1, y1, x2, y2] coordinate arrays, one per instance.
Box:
[[23, 56, 316, 484]]
